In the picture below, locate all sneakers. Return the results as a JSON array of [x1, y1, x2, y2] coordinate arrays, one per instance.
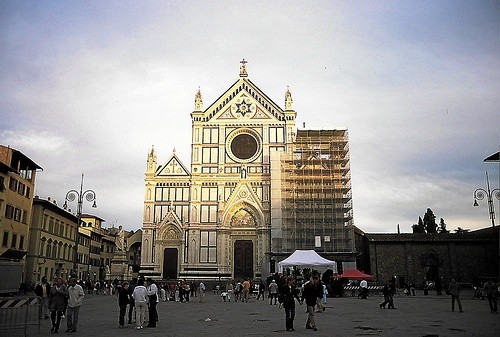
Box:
[[119, 319, 158, 329]]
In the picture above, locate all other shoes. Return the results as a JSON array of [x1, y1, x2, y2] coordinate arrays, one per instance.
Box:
[[51, 328, 55, 331], [306, 326, 312, 328], [313, 327, 318, 331], [53, 330, 58, 333], [65, 328, 72, 332], [70, 329, 76, 332]]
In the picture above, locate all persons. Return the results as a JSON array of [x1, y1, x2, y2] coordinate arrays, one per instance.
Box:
[[350, 280, 358, 296], [360, 278, 368, 299], [49, 277, 69, 333], [473, 277, 500, 313], [395, 276, 447, 296], [279, 274, 303, 332], [65, 276, 84, 333], [379, 282, 390, 309], [34, 276, 51, 320], [216, 272, 329, 313], [119, 283, 131, 329], [127, 276, 207, 329], [388, 280, 396, 309], [300, 272, 324, 331], [51, 276, 123, 319], [449, 276, 464, 313]]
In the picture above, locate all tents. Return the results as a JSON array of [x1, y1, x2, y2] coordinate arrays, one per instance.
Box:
[[338, 269, 374, 280], [278, 249, 335, 278]]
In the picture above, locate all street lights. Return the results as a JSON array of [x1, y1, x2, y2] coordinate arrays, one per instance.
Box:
[[474, 171, 500, 226], [63, 173, 97, 274]]
